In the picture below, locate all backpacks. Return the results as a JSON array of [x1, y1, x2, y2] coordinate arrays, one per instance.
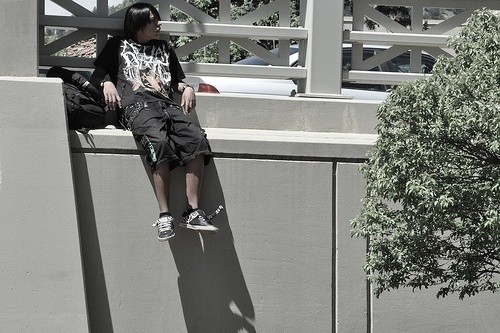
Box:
[[44, 65, 119, 134]]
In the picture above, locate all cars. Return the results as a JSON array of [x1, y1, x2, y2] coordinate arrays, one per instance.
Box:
[[178, 40, 445, 102]]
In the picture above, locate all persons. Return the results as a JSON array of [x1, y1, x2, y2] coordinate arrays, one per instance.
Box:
[[89, 2, 224, 240]]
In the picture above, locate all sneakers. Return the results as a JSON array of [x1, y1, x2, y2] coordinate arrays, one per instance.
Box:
[[152, 216, 175, 241], [178, 209, 220, 234]]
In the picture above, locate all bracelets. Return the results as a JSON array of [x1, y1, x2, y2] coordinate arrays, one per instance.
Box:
[[181, 84, 191, 92]]
